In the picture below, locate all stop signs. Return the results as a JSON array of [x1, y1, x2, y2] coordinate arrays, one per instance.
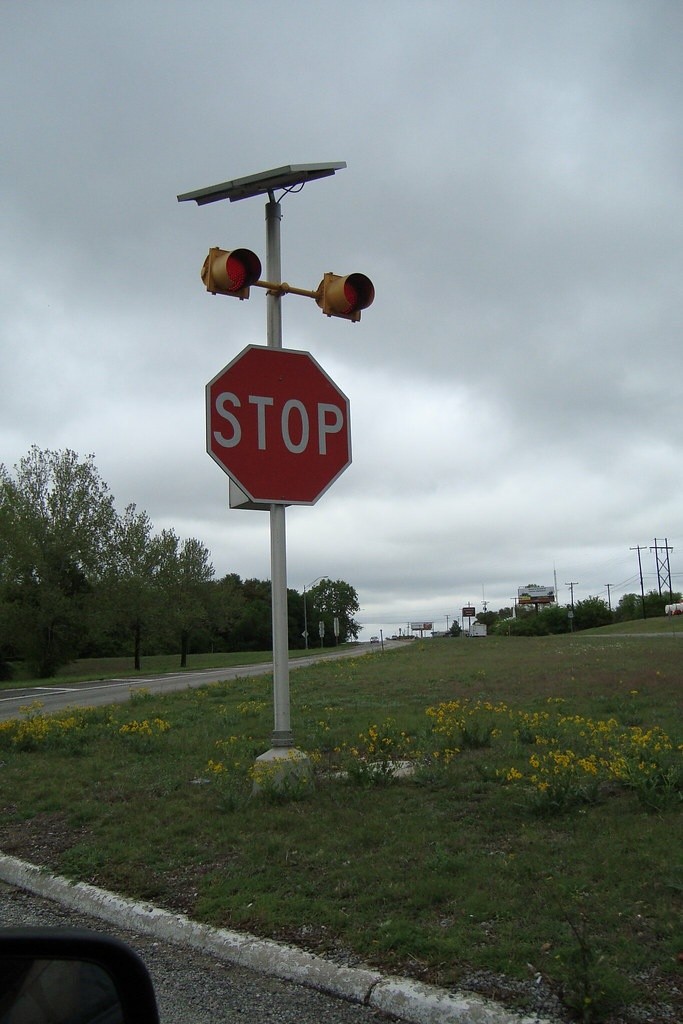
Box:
[[206, 344, 353, 506]]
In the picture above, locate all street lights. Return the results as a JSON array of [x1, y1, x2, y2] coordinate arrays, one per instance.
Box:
[[304, 576, 329, 650]]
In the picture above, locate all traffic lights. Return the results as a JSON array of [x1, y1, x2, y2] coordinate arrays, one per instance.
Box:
[[316, 272, 375, 324], [200, 246, 261, 301]]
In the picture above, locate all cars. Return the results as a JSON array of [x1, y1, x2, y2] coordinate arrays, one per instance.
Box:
[[392, 635, 398, 640], [370, 636, 379, 644]]
